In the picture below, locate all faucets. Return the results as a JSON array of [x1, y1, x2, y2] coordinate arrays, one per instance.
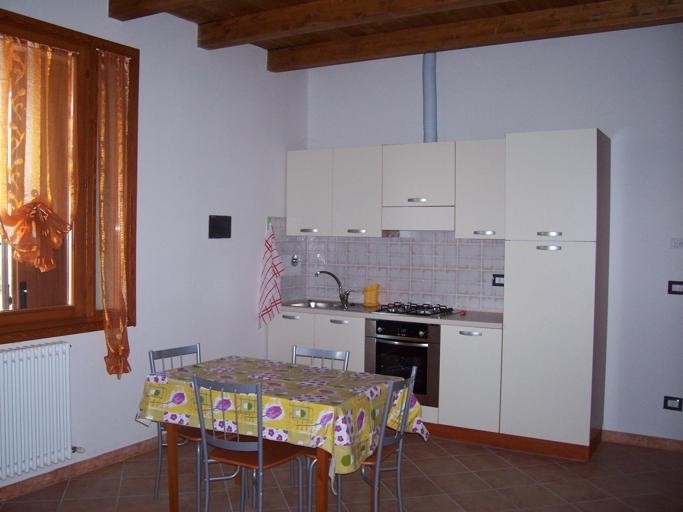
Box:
[[315, 270, 343, 292]]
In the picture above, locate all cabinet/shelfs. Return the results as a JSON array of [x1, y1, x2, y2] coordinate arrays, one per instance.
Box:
[[333, 145, 400, 237], [438, 325, 502, 434], [382, 141, 455, 231], [499, 128, 612, 464], [454, 140, 506, 241], [286, 148, 334, 237], [314, 314, 376, 375], [266, 311, 315, 365]]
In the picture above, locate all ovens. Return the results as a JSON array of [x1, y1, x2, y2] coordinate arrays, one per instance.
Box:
[[365, 336, 440, 409]]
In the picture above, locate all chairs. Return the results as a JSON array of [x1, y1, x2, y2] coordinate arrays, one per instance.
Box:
[[192, 374, 305, 512], [148, 342, 235, 512], [309, 365, 418, 512], [277, 345, 350, 497]]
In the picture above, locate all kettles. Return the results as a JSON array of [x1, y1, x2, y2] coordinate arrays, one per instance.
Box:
[[361, 282, 380, 309]]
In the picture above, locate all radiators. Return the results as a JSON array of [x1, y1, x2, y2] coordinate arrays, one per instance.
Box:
[[1, 340, 75, 482]]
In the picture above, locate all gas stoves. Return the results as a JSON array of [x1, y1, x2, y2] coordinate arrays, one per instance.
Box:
[[375, 302, 462, 337]]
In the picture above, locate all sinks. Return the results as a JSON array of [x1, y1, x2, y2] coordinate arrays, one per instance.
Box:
[[290, 302, 339, 309]]
